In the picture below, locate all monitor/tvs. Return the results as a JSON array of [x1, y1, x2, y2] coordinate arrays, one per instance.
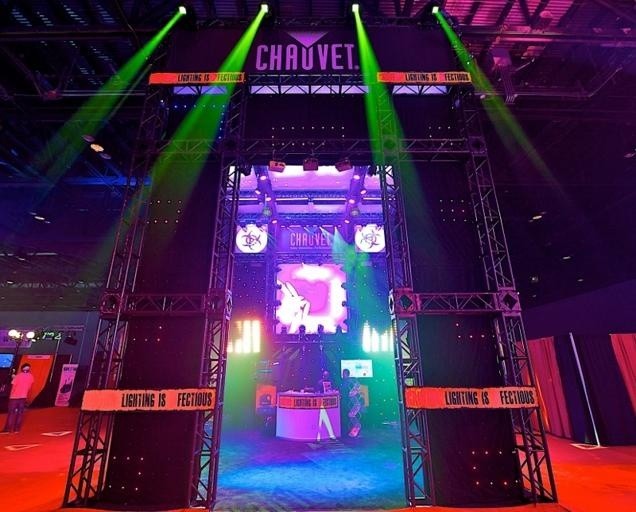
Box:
[[323, 382, 331, 394], [0, 353, 15, 368]]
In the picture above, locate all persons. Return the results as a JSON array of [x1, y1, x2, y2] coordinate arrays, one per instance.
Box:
[[0, 363, 34, 435], [315, 367, 354, 394]]
